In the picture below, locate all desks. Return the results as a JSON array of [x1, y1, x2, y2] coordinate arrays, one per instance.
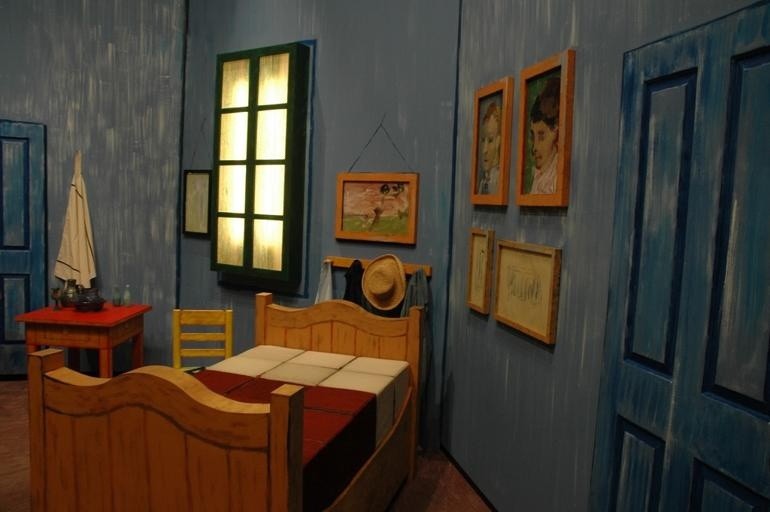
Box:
[[15, 301, 153, 379]]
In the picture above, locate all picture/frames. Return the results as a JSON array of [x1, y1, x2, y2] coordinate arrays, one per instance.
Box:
[[335, 171, 418, 245], [467, 48, 576, 345], [182, 169, 212, 236]]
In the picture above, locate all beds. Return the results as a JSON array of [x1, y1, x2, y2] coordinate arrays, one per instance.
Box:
[[27, 291, 425, 512]]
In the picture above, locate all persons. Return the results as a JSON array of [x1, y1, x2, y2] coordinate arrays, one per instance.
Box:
[[346, 182, 409, 231], [477, 100, 501, 194], [527, 76, 560, 194]]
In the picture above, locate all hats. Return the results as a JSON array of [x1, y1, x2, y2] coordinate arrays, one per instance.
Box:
[[361, 253, 406, 311]]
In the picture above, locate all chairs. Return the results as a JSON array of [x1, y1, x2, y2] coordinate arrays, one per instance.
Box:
[[171, 307, 235, 372]]
